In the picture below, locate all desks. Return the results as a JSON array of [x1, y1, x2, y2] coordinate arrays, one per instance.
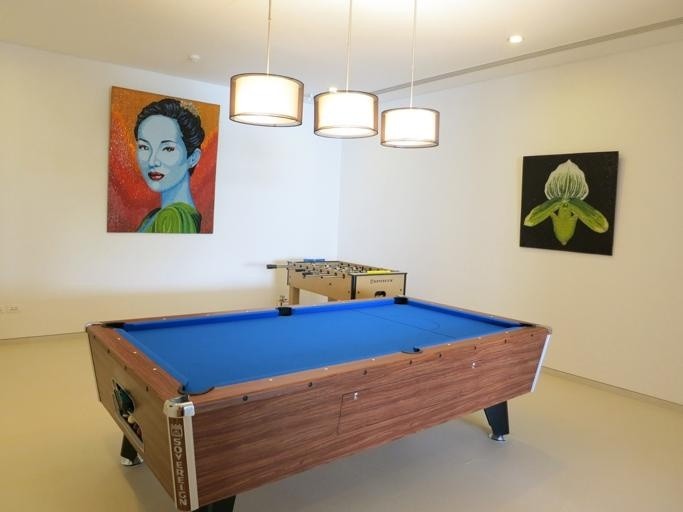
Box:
[[84, 296, 554, 511]]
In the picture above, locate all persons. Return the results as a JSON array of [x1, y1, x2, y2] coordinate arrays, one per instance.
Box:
[[134, 98, 205, 234]]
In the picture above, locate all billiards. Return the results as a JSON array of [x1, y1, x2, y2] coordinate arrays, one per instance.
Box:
[[122, 413, 143, 440]]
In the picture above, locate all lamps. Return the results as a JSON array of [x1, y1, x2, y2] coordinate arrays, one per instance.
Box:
[[228, 2, 440, 148]]
